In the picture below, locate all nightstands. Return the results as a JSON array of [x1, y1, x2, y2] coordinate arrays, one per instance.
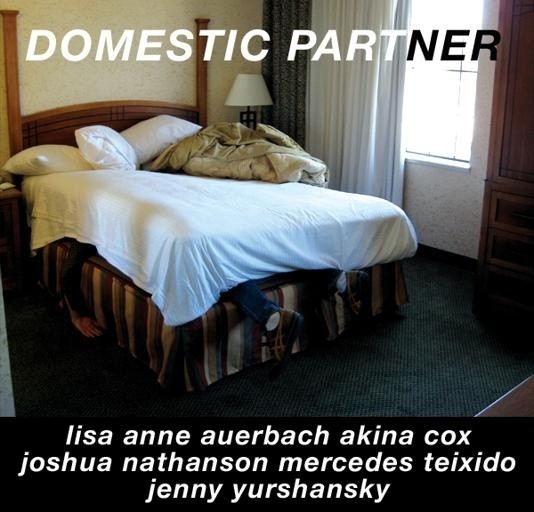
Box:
[[1, 180, 25, 291]]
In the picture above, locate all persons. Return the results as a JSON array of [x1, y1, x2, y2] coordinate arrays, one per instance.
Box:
[[59, 236, 371, 371]]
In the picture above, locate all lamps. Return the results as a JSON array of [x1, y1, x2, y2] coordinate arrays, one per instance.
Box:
[[223, 75, 275, 134]]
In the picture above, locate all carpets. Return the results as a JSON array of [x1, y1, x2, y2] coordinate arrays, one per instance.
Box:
[[0, 243, 534, 509]]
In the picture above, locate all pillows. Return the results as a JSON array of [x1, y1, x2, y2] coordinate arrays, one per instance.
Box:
[[2, 115, 203, 173]]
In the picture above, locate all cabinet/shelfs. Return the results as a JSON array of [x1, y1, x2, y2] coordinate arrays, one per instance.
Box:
[[471, 0, 534, 327]]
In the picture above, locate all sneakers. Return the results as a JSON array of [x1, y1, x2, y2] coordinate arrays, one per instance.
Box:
[[335, 269, 370, 338], [265, 306, 305, 375]]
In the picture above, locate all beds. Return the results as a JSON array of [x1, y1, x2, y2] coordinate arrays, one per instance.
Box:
[[18, 101, 408, 385]]
